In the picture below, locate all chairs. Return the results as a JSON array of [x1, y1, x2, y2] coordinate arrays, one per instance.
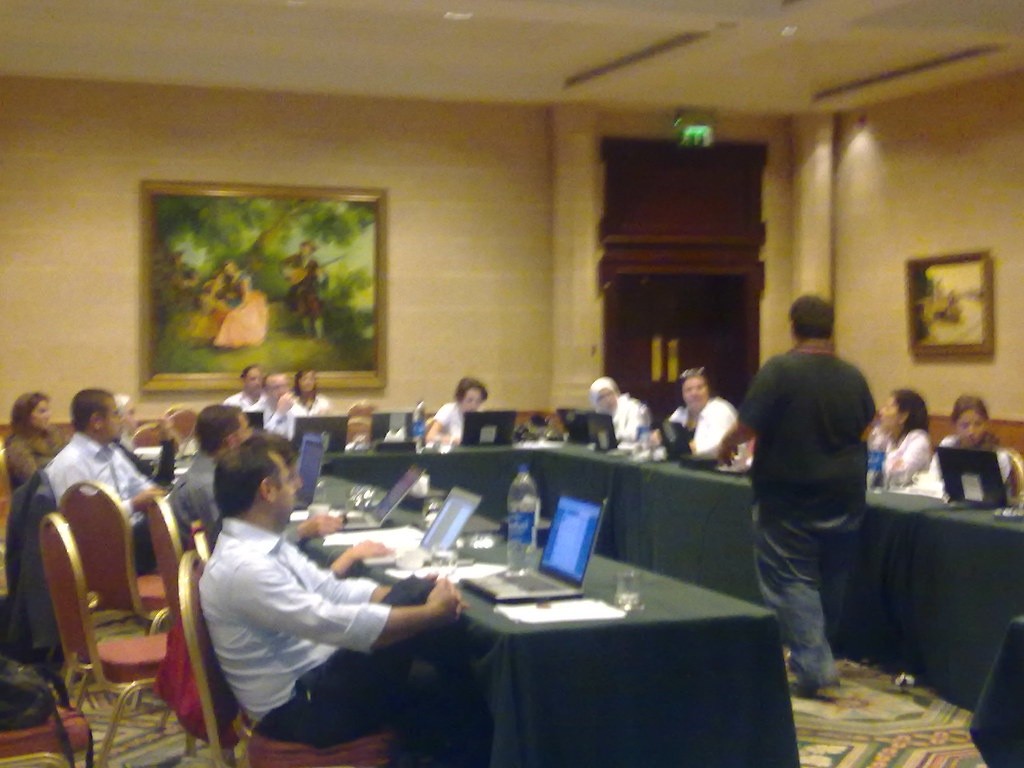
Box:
[[176, 547, 409, 768], [1, 404, 216, 768]]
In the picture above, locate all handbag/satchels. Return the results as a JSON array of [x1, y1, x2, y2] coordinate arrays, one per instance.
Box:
[[1, 659, 56, 728]]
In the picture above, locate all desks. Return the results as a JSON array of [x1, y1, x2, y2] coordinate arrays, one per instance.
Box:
[[246, 436, 1023, 768]]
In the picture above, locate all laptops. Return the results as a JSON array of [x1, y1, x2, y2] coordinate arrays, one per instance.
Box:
[[239, 409, 694, 605], [933, 446, 1019, 509]]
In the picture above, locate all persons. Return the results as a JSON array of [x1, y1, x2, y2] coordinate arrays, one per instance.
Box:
[[212, 431, 393, 578], [925, 394, 1015, 500], [170, 406, 253, 548], [651, 367, 747, 463], [736, 295, 874, 697], [112, 396, 177, 488], [40, 390, 169, 575], [286, 371, 330, 418], [588, 377, 650, 445], [423, 377, 488, 443], [196, 442, 471, 749], [220, 366, 269, 412], [246, 373, 309, 440], [868, 390, 934, 487], [8, 393, 62, 484]]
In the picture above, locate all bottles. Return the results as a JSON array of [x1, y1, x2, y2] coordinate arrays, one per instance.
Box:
[[507, 463, 540, 578], [411, 397, 425, 454], [865, 419, 884, 492]]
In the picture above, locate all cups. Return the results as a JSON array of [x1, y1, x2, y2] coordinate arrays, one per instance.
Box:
[[350, 485, 373, 508], [409, 472, 432, 497], [423, 490, 445, 529], [440, 434, 454, 453], [889, 461, 910, 491], [616, 572, 647, 609]]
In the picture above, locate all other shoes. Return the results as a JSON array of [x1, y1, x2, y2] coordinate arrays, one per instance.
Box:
[[792, 679, 842, 700]]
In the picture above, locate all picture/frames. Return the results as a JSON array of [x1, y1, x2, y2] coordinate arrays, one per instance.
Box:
[[904, 251, 996, 359], [137, 178, 392, 394]]
